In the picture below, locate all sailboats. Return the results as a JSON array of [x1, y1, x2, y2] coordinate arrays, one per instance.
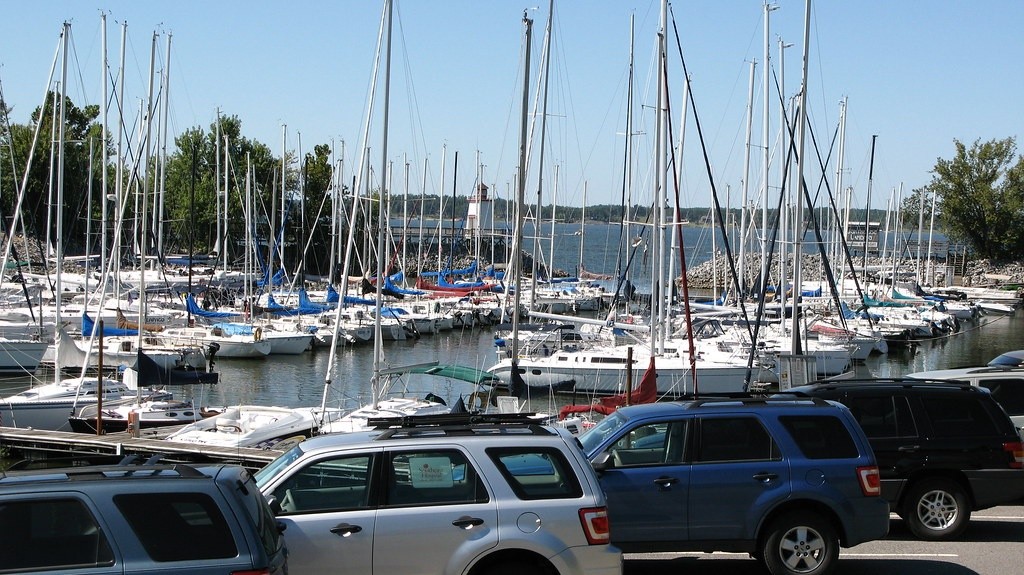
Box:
[[2, 0, 1024, 464]]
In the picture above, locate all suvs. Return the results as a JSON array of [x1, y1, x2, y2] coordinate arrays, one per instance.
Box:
[[0, 460, 292, 574], [452, 390, 891, 575], [89, 411, 627, 575], [629, 378, 1024, 542]]
